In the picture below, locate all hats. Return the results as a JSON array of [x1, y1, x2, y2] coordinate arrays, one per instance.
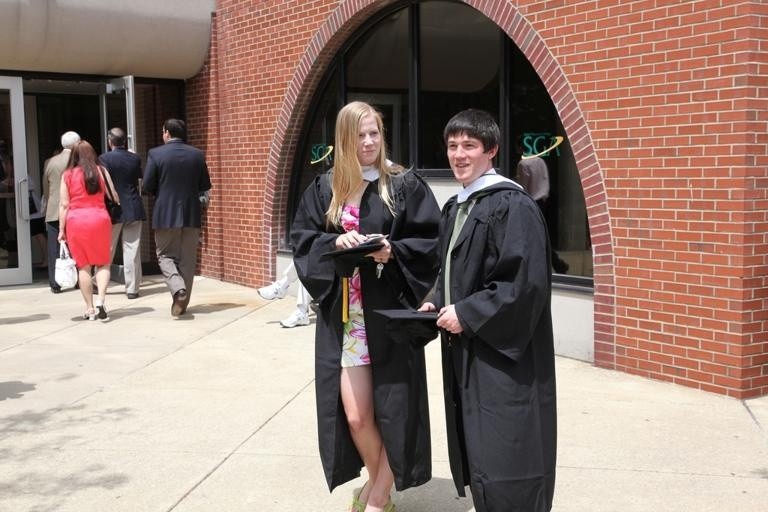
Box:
[[374, 309, 458, 405], [322, 243, 385, 323]]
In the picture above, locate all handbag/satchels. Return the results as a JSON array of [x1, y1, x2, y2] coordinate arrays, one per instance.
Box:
[[100, 166, 122, 224], [54, 239, 78, 291]]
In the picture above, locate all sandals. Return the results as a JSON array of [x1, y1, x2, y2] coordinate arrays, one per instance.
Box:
[[352, 481, 394, 512]]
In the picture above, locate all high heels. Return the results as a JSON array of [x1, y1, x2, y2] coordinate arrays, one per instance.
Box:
[[82, 308, 95, 320], [96, 300, 107, 319]]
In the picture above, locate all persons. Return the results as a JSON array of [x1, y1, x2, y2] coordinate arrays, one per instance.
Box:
[[1, 117, 146, 321], [289, 101, 439, 510], [511, 136, 569, 277], [417, 109, 551, 510], [141, 118, 212, 317]]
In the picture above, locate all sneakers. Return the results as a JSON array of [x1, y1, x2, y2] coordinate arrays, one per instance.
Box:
[[258, 282, 287, 299], [280, 312, 310, 327]]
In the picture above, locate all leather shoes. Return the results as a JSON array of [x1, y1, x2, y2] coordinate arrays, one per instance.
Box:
[[171, 289, 187, 316]]
